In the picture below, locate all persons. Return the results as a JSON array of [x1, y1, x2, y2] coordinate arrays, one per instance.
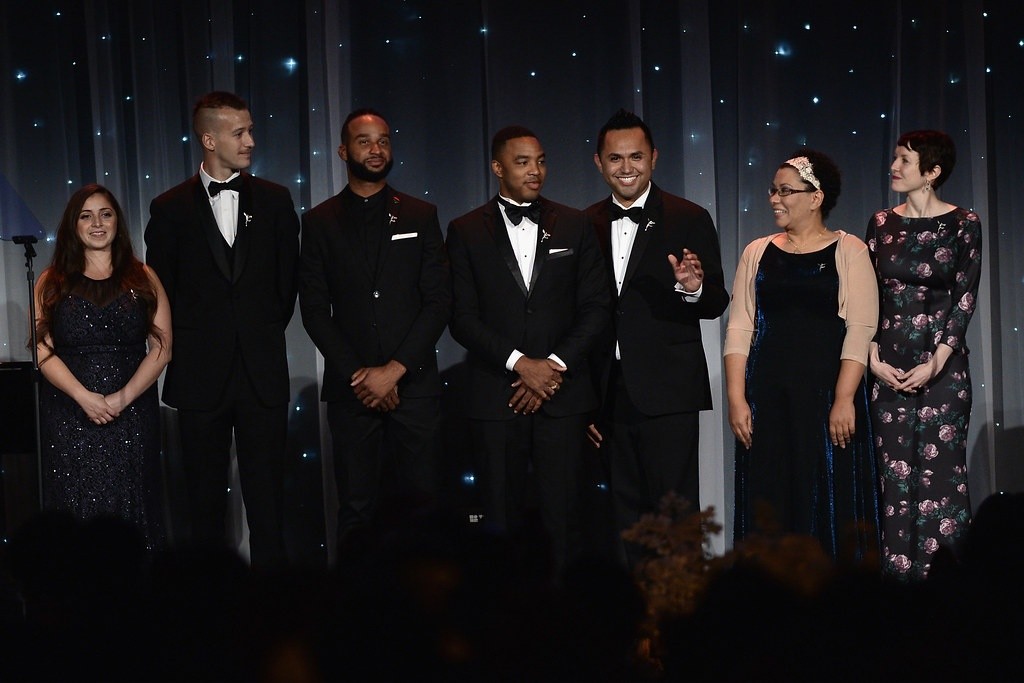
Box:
[[582, 108, 730, 595], [0, 492, 1024, 683], [723, 147, 879, 589], [864, 129, 983, 581], [28, 183, 172, 571], [445, 126, 609, 596], [298, 106, 450, 597], [145, 91, 299, 595]]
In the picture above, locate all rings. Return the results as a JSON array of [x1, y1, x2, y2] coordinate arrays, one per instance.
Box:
[[551, 383, 558, 390]]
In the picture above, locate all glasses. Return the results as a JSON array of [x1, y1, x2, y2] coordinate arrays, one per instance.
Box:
[[768, 186, 813, 198]]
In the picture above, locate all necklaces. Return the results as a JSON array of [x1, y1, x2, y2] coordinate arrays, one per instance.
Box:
[[786, 227, 828, 254]]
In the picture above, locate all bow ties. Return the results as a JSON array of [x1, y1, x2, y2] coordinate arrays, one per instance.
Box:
[[209, 176, 240, 197], [495, 194, 540, 225], [610, 203, 643, 224]]
